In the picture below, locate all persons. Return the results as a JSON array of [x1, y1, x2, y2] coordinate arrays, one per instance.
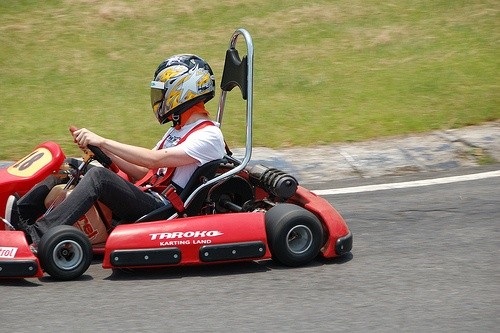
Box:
[[4, 54, 227, 257]]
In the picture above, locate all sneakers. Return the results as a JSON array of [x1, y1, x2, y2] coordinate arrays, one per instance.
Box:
[[5, 195, 40, 258]]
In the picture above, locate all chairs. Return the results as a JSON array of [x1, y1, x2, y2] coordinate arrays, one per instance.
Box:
[[135, 154, 228, 224]]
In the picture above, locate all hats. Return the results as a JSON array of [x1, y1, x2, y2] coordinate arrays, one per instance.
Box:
[[151, 54, 215, 124]]
